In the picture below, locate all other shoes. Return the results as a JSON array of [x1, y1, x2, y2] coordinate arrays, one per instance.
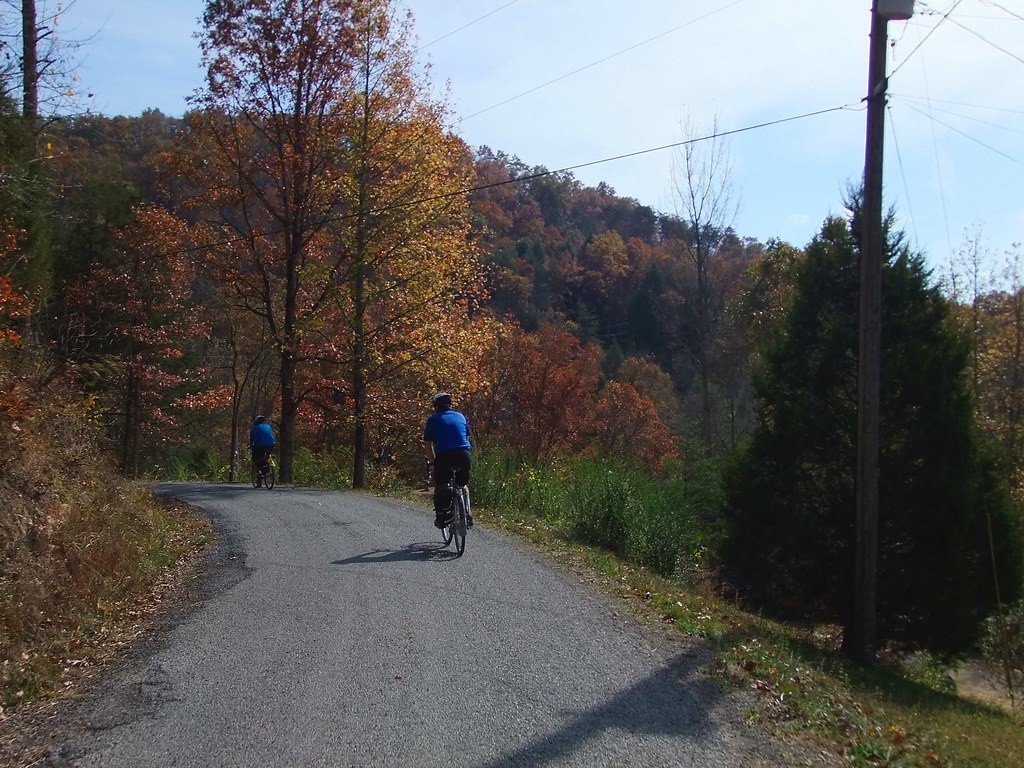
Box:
[[467, 511, 473, 526], [434, 513, 454, 528]]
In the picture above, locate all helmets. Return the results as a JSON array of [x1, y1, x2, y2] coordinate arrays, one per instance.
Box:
[[430, 392, 453, 403], [254, 415, 264, 421]]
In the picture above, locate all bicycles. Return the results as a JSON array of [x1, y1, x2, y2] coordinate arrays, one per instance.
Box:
[[422, 453, 472, 555], [245, 443, 277, 490]]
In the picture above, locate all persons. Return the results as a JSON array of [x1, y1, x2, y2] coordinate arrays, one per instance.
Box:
[[249, 414, 275, 478], [424, 392, 470, 526]]
